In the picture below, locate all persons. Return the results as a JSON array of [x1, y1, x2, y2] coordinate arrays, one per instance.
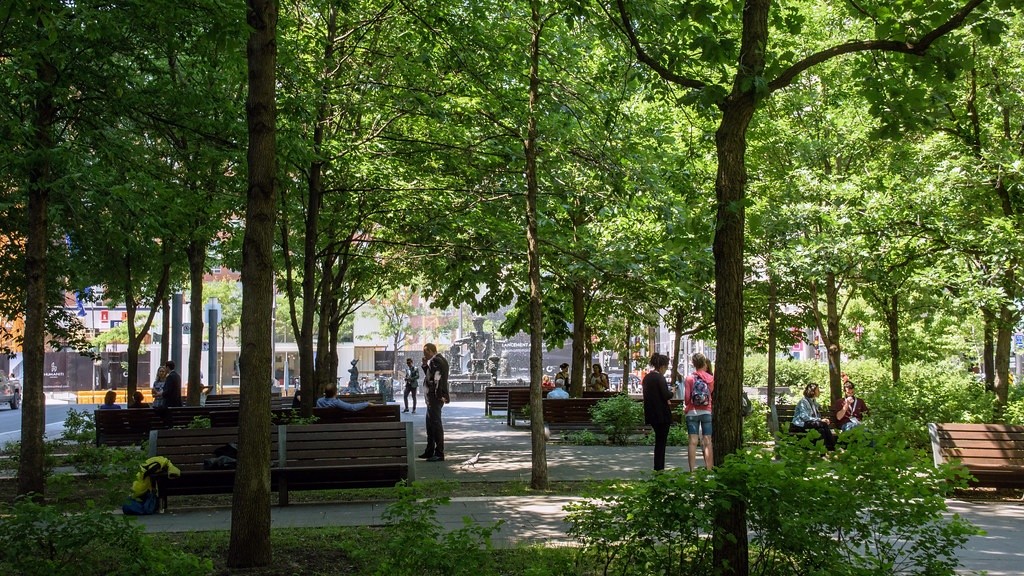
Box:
[[315, 383, 374, 411], [833, 381, 871, 431], [291, 390, 301, 408], [685, 354, 714, 472], [704, 358, 713, 376], [543, 375, 569, 399], [9, 373, 16, 380], [643, 353, 675, 478], [788, 384, 837, 453], [128, 392, 149, 408], [199, 384, 213, 406], [100, 390, 121, 410], [401, 358, 419, 414], [151, 361, 183, 407], [587, 364, 609, 392], [555, 363, 570, 386], [418, 343, 450, 463], [672, 372, 686, 400]]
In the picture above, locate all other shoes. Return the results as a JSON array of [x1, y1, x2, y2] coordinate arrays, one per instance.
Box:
[[402, 409, 409, 413], [427, 456, 444, 461], [418, 454, 430, 458], [412, 408, 416, 413]]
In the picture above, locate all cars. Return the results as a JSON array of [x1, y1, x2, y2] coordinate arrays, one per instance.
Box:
[[367, 378, 402, 394]]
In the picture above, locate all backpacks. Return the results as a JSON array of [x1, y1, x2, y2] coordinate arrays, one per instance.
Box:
[[691, 373, 709, 406]]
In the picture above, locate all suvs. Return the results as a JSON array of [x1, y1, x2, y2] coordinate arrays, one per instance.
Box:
[[0, 369, 22, 410]]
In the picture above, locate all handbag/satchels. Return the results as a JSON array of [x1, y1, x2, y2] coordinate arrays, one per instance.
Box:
[[122, 494, 159, 514]]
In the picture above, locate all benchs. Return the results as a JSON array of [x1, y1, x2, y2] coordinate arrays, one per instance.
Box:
[[485, 387, 933, 461], [96, 392, 415, 513], [928, 422, 1024, 502]]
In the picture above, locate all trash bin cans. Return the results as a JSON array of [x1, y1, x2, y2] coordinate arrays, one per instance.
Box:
[[379, 378, 394, 401]]
